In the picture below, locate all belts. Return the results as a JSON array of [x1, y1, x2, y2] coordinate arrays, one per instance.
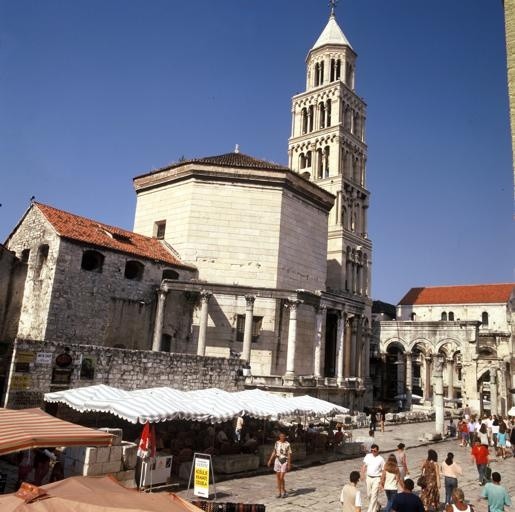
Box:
[[368, 475, 382, 478]]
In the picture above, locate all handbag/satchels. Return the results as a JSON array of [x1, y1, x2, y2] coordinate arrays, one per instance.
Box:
[[280, 457, 287, 464], [485, 468, 491, 480], [417, 476, 427, 488], [404, 475, 411, 481]]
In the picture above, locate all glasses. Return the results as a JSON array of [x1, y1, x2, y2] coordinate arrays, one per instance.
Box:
[[371, 449, 377, 452]]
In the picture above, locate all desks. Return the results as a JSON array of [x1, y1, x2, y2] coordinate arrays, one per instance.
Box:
[[166, 425, 347, 479]]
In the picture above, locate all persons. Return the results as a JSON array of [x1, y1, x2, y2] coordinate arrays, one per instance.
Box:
[[120, 412, 340, 464], [267, 431, 292, 498], [339, 404, 515, 512], [31, 446, 56, 487], [14, 448, 38, 490]]
